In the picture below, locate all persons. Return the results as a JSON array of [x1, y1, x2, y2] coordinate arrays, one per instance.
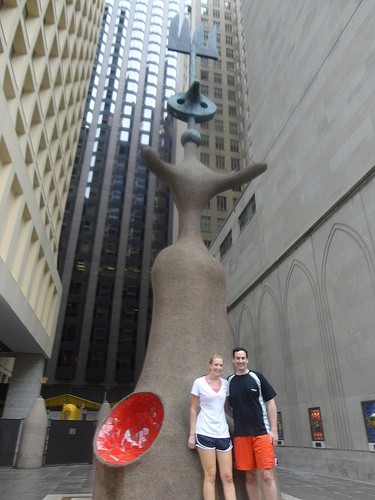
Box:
[[187, 355, 236, 500], [226, 347, 279, 500]]
